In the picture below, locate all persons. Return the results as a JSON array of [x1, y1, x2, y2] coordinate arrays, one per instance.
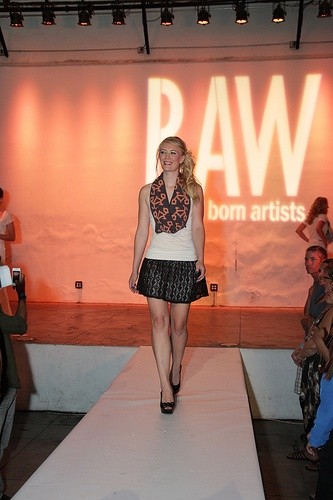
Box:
[[0, 188, 29, 500], [288, 245, 333, 500], [295, 197, 333, 250], [128, 137, 209, 415]]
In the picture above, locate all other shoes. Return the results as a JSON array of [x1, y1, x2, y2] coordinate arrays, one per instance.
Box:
[[287, 451, 319, 462], [305, 463, 321, 472]]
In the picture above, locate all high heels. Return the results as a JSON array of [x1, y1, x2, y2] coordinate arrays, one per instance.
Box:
[[160, 390, 175, 414], [169, 364, 183, 393]]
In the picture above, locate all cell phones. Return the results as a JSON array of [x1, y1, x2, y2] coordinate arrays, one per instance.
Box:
[[11, 268, 22, 285]]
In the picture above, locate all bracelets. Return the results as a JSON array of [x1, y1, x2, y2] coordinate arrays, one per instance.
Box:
[[18, 296, 26, 300]]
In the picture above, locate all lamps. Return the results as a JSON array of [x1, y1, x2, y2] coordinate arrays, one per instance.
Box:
[[41, 3, 57, 25], [197, 5, 211, 25], [234, 0, 248, 23], [161, 7, 174, 25], [8, 2, 25, 27], [318, 0, 332, 18], [112, 1, 126, 25], [77, 1, 93, 25], [272, 0, 286, 23]]
